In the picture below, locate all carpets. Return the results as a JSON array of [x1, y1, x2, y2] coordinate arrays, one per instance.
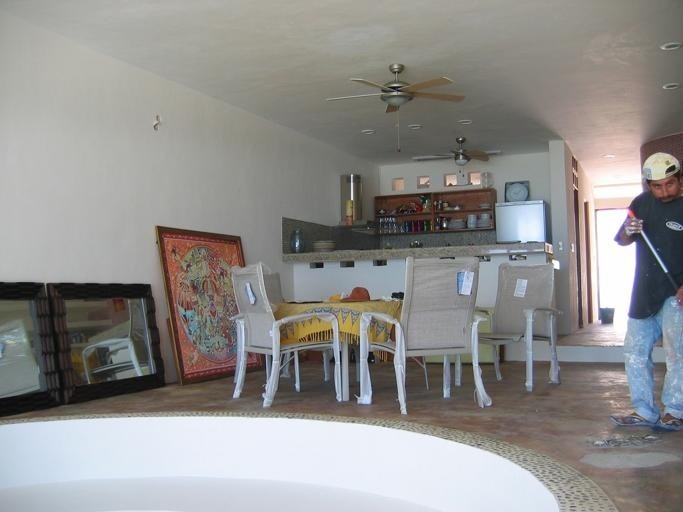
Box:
[[0, 412, 618, 510]]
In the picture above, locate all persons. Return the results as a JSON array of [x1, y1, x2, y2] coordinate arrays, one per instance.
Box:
[[606, 150, 683, 432]]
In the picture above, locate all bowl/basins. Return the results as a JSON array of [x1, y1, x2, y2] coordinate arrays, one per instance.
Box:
[[466, 214, 476, 228], [480, 213, 490, 219], [311, 240, 336, 252]]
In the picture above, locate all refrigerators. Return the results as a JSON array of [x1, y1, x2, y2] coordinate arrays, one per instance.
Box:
[[494, 200, 546, 243]]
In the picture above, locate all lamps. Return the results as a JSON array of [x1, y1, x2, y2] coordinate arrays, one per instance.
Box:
[[385, 96, 409, 152], [454, 160, 468, 167]]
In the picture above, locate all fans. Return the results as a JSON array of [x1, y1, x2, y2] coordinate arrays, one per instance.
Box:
[[327, 63, 466, 113], [433, 137, 490, 166]]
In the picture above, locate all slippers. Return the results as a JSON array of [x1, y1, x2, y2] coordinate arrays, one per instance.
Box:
[[610, 415, 657, 426], [657, 414, 683, 431]]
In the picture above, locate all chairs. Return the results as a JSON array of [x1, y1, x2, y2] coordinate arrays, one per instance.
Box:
[[231, 264, 342, 407], [81, 299, 143, 382], [357, 256, 492, 414], [227, 265, 293, 381], [454, 262, 564, 391]]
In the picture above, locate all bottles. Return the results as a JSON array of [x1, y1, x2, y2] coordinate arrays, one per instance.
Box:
[[378, 217, 451, 234], [422, 199, 448, 211]]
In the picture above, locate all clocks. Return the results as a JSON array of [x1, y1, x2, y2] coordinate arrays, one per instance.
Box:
[[505, 180, 530, 201]]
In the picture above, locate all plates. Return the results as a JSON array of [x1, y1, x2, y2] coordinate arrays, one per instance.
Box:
[[478, 204, 491, 207], [476, 217, 491, 227], [448, 218, 465, 229]]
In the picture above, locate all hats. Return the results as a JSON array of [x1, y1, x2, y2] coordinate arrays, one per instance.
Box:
[[340, 287, 370, 302], [642, 152, 681, 181]]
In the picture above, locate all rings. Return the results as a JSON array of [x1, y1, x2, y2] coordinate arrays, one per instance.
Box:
[[678, 299, 681, 303]]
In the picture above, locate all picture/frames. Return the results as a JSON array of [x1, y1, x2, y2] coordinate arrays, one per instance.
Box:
[[154, 225, 264, 386], [45, 282, 165, 406], [1, 282, 63, 417]]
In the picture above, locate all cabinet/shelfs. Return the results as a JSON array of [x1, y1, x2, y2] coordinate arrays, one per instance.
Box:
[[373, 189, 496, 236]]
